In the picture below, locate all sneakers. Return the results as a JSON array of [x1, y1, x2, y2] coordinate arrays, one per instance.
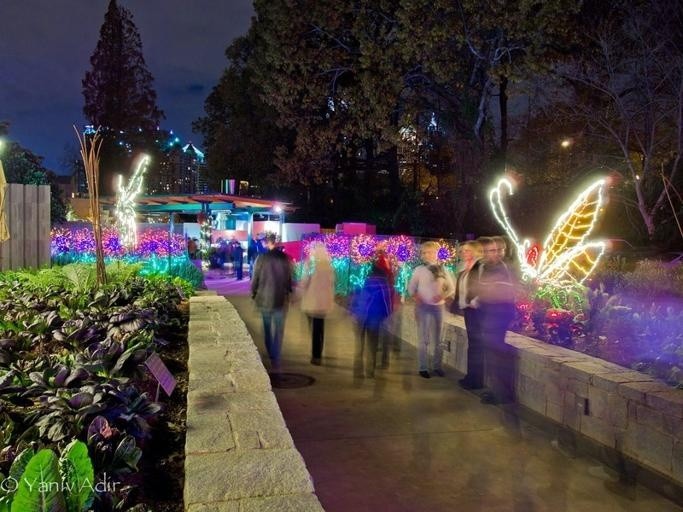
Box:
[[459, 379, 479, 390], [420, 371, 430, 378], [434, 369, 445, 377]]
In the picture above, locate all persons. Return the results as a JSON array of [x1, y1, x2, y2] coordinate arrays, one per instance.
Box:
[[295, 235, 515, 405], [187, 232, 268, 280], [250, 234, 293, 367]]
